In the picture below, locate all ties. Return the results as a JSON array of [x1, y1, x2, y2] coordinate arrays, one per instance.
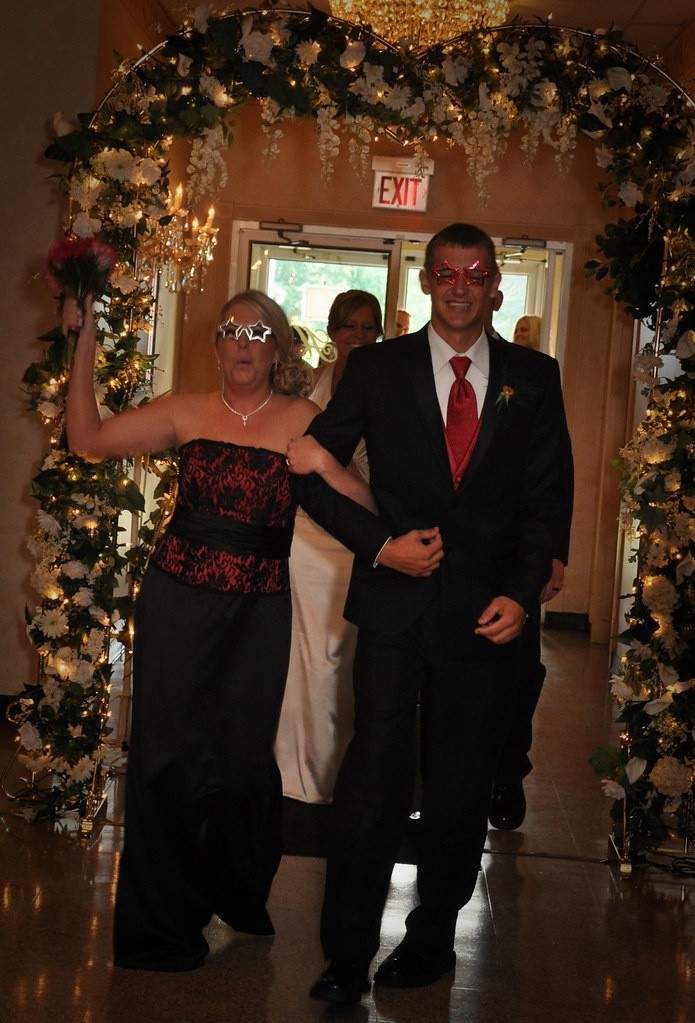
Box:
[[445, 356, 479, 491]]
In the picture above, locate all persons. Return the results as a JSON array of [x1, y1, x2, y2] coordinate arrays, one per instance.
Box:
[[58, 291, 378, 970], [274, 289, 386, 805], [383, 310, 414, 339], [514, 316, 543, 349], [490, 443, 576, 830], [284, 326, 308, 359], [292, 224, 564, 1001]]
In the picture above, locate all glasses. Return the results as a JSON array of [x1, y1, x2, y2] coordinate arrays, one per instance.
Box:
[[217, 315, 277, 342], [432, 259, 496, 286]]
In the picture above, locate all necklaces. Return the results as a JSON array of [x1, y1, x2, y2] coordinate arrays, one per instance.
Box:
[[221, 389, 274, 426]]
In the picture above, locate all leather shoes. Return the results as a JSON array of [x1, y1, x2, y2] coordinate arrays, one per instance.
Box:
[[490, 781, 527, 829], [310, 956, 371, 1002], [375, 941, 456, 988]]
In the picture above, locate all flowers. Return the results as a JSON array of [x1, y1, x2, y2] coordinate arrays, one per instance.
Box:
[[496, 386, 513, 415], [0, 0, 695, 874]]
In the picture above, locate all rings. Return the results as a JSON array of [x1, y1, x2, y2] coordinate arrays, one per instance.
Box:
[[286, 459, 291, 466], [552, 587, 560, 591]]
